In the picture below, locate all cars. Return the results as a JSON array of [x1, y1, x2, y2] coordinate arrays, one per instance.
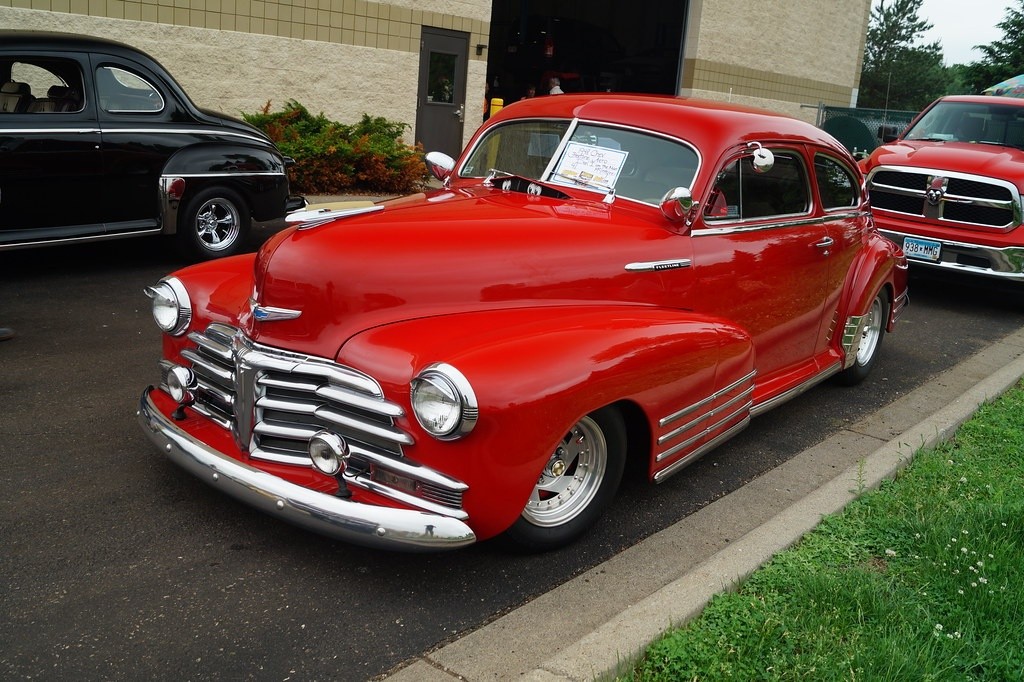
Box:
[[1, 27, 311, 269], [851, 93, 1024, 286], [136, 91, 911, 554]]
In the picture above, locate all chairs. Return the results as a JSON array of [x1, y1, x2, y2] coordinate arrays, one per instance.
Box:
[[0, 82, 37, 112], [27, 86, 80, 112], [676, 173, 781, 217]]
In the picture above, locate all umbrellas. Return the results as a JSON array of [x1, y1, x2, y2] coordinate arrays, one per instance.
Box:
[[980, 73, 1024, 99]]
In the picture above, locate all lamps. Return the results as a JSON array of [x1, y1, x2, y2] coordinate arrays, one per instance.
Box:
[[543, 17, 554, 58]]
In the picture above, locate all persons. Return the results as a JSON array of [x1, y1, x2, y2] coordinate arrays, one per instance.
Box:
[[484, 83, 489, 115], [549, 78, 564, 96], [521, 85, 537, 100]]
